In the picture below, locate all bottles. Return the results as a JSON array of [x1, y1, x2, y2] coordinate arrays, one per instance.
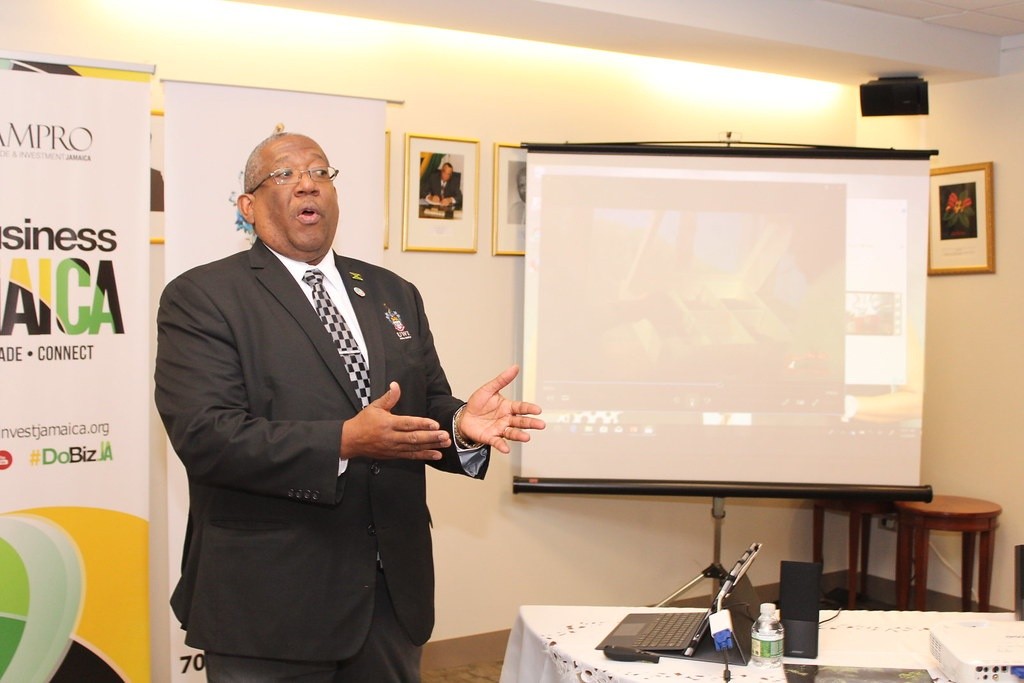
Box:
[[750, 602, 786, 683]]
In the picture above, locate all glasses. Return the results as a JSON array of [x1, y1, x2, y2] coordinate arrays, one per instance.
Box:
[[248, 167, 339, 195]]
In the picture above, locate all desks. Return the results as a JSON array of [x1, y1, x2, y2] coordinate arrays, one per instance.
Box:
[[497, 605, 1024, 683]]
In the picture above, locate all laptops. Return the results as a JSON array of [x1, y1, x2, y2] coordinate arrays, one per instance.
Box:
[[595, 543, 757, 656]]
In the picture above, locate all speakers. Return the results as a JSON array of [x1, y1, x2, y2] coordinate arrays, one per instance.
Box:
[[860, 76, 929, 117], [779, 560, 821, 658]]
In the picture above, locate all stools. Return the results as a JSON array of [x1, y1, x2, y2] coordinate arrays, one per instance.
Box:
[[811, 493, 1003, 613]]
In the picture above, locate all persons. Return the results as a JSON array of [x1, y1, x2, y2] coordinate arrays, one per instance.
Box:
[[423, 163, 462, 206], [509, 167, 526, 224], [154, 132, 547, 683]]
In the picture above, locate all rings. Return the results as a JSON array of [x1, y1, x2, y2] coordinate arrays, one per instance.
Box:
[[502, 427, 511, 435]]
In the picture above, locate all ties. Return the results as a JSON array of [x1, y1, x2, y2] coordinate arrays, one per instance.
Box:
[[302, 268, 371, 412]]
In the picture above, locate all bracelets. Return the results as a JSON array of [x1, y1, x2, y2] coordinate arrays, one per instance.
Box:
[[454, 409, 484, 449]]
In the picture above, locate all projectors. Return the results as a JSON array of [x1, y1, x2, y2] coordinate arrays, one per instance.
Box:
[[927, 623, 1024, 683]]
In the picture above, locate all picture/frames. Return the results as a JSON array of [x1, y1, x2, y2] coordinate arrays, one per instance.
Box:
[[401, 131, 480, 256], [926, 161, 998, 276], [492, 141, 530, 258]]
